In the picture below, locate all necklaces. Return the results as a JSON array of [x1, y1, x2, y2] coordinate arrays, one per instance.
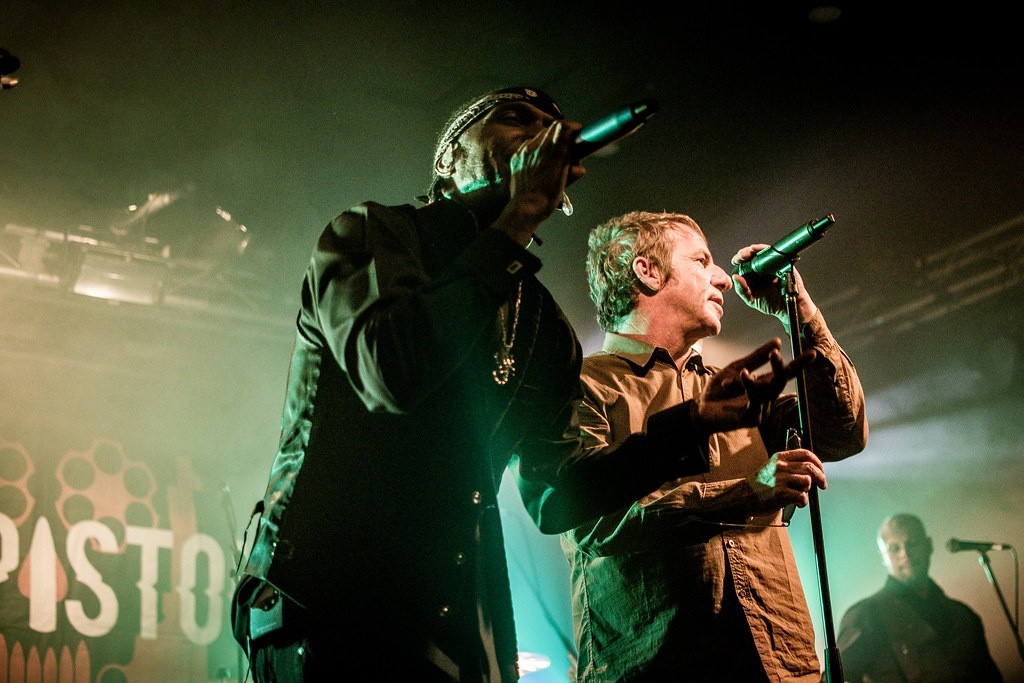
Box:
[[490, 282, 521, 385]]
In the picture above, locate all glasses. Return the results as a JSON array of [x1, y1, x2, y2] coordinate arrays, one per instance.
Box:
[[693, 427, 802, 527]]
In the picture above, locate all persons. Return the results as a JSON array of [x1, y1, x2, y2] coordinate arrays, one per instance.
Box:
[[837, 514, 1004, 683], [519, 210, 870, 683], [231, 89, 818, 683]]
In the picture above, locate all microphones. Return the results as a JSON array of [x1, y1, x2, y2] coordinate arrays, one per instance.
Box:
[[945, 537, 1011, 552], [732, 215, 836, 291], [218, 480, 232, 503], [569, 103, 657, 169]]
